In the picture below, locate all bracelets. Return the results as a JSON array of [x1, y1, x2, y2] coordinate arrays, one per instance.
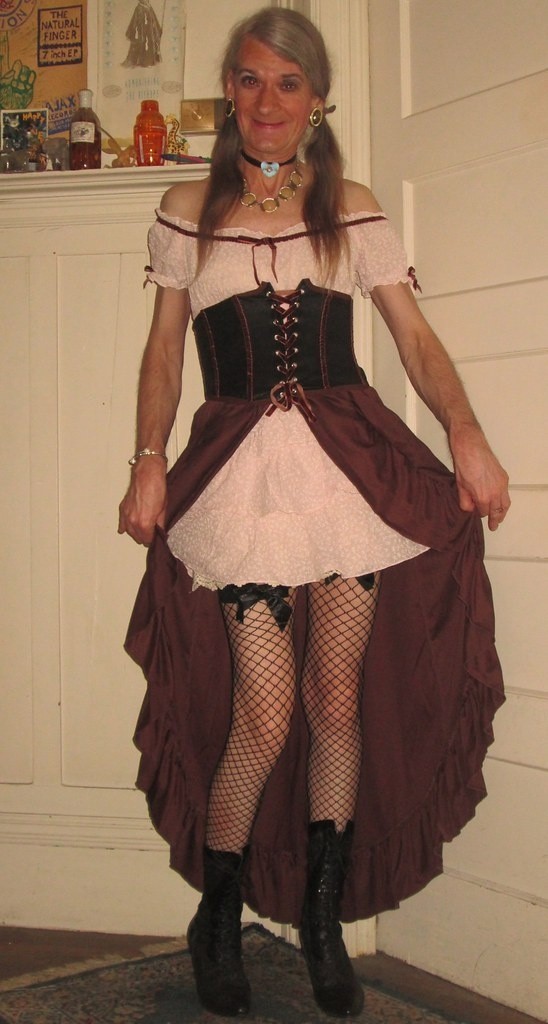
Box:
[[129, 449, 168, 464]]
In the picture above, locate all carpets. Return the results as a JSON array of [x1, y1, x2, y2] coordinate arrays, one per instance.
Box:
[[1, 921, 468, 1024]]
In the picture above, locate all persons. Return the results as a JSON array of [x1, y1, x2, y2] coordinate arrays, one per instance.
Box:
[[119, 7, 511, 1019]]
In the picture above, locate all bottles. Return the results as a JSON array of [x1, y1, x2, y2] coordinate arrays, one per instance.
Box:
[[134, 101, 167, 166], [69, 88, 101, 170]]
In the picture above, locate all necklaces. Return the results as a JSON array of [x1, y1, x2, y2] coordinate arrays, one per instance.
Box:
[[240, 161, 303, 212], [241, 149, 298, 176]]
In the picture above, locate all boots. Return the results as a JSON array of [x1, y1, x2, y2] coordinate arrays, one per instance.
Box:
[[298, 819, 365, 1019], [186, 843, 252, 1019]]
